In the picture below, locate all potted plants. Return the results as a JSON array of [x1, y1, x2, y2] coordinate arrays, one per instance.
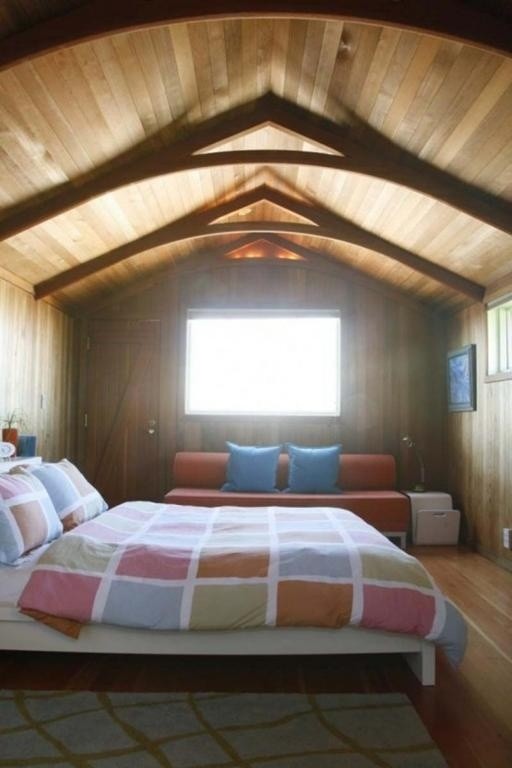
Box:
[[0, 404, 36, 458]]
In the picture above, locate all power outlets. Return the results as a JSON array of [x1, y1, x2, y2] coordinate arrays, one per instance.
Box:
[[501, 527, 511, 550]]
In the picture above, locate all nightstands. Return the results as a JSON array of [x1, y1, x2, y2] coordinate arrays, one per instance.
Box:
[[402, 489, 462, 546]]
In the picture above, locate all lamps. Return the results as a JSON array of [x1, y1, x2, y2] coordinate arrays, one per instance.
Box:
[[401, 431, 427, 493]]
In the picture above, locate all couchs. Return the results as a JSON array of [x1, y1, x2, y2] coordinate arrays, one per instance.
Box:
[[161, 452, 413, 550]]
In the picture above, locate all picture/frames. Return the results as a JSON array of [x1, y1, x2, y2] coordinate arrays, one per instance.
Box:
[[445, 345, 479, 415]]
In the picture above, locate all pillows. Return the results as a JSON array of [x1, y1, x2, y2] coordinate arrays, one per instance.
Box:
[[280, 441, 346, 495], [221, 439, 282, 494], [21, 457, 110, 533], [2, 467, 65, 568]]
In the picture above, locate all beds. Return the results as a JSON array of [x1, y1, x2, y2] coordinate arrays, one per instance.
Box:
[[1, 458, 440, 689]]
